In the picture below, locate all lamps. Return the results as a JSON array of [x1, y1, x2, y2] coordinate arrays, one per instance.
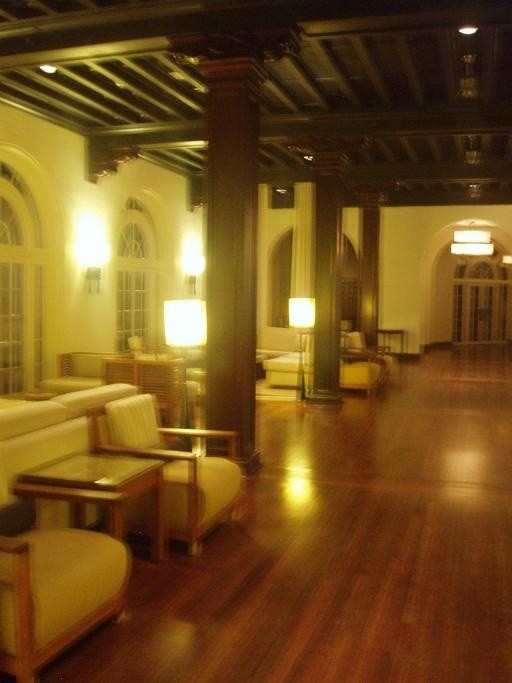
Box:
[[450, 25, 495, 258], [180, 229, 207, 293], [72, 210, 115, 297], [286, 297, 313, 399], [160, 300, 209, 379]]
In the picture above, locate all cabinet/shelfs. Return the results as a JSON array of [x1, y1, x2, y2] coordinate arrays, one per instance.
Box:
[[100, 356, 188, 450]]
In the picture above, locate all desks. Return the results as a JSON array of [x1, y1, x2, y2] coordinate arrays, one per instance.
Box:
[[7, 448, 170, 577], [24, 389, 58, 403]]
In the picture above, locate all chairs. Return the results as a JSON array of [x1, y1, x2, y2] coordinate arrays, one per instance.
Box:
[[0, 515, 132, 683], [86, 393, 242, 560], [256, 325, 393, 404]]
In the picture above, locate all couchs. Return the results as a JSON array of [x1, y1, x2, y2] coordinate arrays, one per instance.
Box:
[[38, 350, 131, 396], [0, 380, 143, 532]]
